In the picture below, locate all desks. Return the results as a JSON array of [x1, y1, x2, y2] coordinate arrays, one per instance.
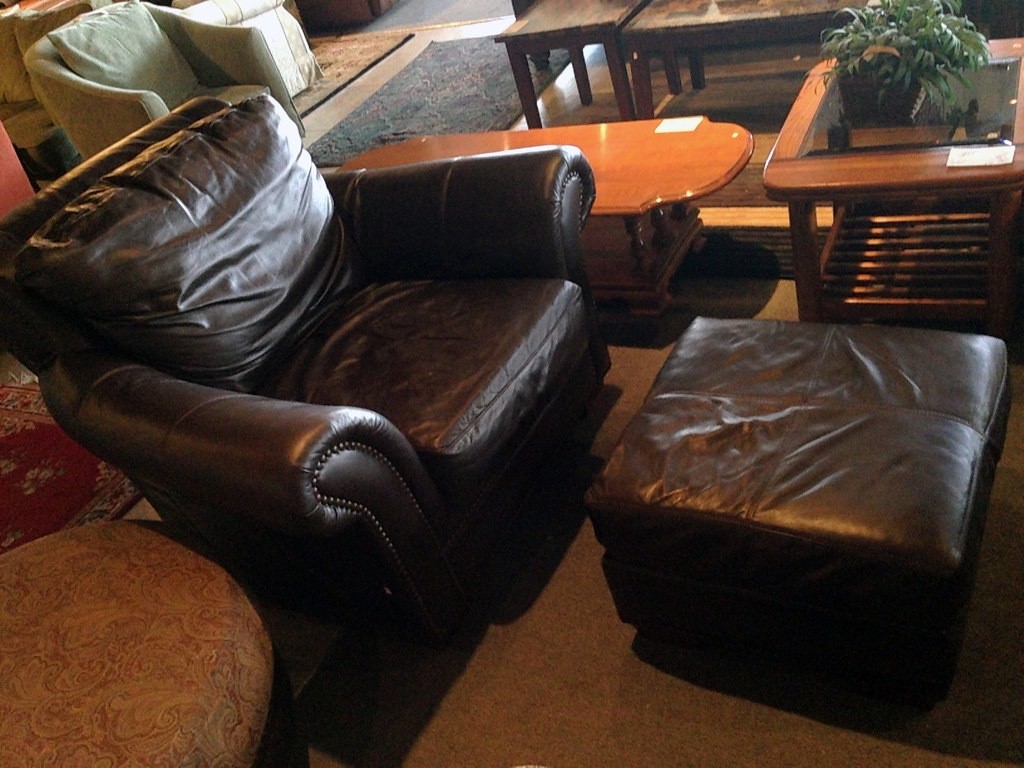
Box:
[[338, 0, 1024, 362]]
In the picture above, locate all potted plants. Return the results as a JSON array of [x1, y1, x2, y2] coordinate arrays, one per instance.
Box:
[[799, 0, 992, 123]]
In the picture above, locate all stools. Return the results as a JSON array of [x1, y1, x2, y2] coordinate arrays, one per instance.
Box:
[[586, 316, 1011, 691]]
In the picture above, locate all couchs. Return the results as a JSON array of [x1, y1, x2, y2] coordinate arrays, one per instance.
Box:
[[0, 2, 612, 622]]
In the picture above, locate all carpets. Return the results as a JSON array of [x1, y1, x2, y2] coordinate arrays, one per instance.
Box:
[[293, 34, 414, 116], [0, 383, 143, 554], [305, 34, 589, 168]]
